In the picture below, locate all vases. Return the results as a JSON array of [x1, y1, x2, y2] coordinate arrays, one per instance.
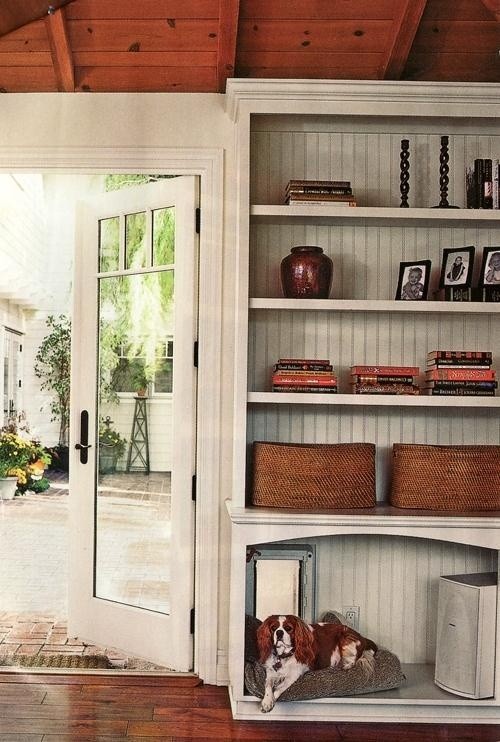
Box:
[[0, 477, 19, 501], [99, 456, 118, 474]]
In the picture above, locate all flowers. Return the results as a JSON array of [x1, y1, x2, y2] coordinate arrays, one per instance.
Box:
[[0, 433, 25, 477], [99, 430, 127, 455]]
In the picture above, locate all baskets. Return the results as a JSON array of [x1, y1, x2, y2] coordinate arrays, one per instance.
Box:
[[252, 440, 375, 511], [389, 440, 500, 514]]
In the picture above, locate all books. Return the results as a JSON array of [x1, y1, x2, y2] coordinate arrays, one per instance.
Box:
[[283, 180, 358, 207], [465, 158, 499, 210], [270, 349, 498, 396]]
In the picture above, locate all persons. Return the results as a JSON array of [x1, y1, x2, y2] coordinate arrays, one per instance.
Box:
[[446, 256, 466, 282], [486, 252, 500, 283], [402, 268, 424, 300]]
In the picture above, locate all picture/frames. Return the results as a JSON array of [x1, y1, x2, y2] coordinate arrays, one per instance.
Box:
[[440, 246, 476, 290], [478, 246, 500, 291], [395, 259, 431, 301]]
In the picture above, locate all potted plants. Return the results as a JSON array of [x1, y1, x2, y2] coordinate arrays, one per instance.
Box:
[[134, 376, 151, 396]]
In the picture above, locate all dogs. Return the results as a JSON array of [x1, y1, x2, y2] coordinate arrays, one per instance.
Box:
[[253, 613, 379, 713]]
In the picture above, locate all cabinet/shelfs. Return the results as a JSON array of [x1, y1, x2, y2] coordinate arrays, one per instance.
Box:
[[226, 71, 499, 733]]
